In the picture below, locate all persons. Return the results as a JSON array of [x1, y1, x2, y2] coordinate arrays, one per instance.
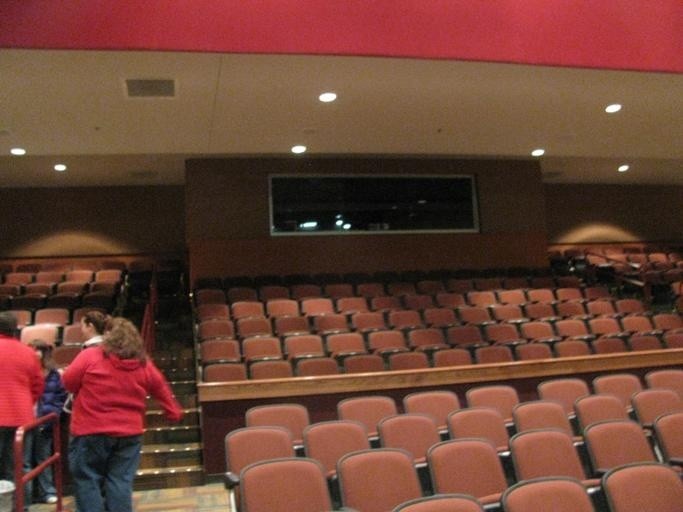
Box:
[[0, 310, 108, 511], [60, 316, 185, 511]]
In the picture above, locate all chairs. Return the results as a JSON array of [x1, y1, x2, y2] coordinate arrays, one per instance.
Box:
[[195, 239, 683, 385], [223, 367, 683, 510]]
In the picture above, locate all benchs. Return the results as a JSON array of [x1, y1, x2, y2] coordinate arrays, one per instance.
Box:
[[0, 257, 129, 370]]
